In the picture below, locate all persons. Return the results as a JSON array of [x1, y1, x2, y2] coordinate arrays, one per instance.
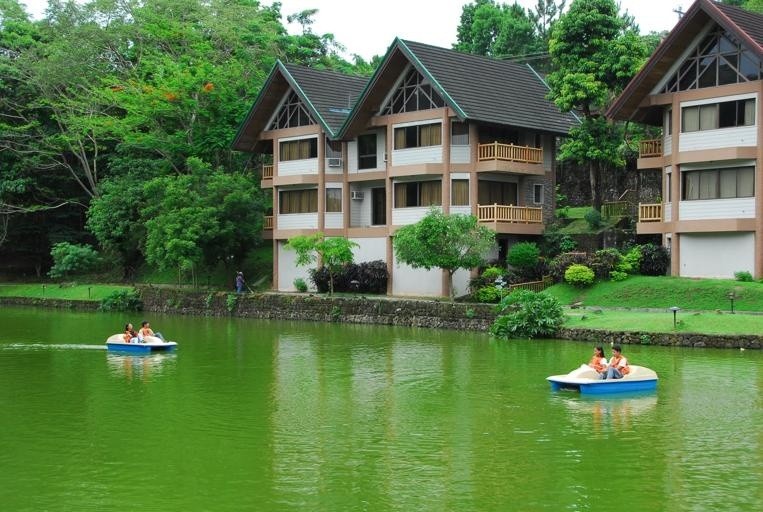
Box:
[[138, 320, 166, 342], [123, 322, 138, 344], [235, 271, 244, 293], [586, 346, 607, 372], [598, 346, 629, 380]]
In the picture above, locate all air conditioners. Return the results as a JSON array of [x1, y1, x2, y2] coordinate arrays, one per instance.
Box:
[[382, 152, 387, 162], [350, 190, 364, 200], [327, 158, 340, 167]]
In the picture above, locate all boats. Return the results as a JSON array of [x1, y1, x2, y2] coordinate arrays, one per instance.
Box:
[[102, 330, 180, 355], [554, 393, 660, 419], [545, 362, 663, 396], [104, 351, 176, 366]]
